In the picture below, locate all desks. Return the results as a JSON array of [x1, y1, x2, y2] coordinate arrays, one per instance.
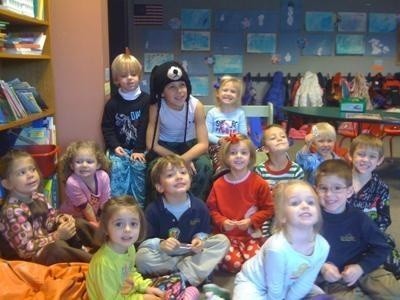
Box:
[[278, 103, 400, 141]]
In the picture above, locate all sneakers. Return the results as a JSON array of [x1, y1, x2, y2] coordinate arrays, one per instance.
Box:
[[203, 283, 231, 300]]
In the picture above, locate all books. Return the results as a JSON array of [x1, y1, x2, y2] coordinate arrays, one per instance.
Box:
[[0, 0, 57, 145]]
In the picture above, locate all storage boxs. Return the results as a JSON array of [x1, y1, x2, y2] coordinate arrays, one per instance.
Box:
[[7, 144, 59, 178]]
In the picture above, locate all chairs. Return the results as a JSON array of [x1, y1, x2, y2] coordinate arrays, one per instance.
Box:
[[381, 122, 399, 158], [337, 120, 358, 153]]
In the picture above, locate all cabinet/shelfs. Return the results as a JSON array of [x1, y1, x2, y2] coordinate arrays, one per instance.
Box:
[[0, 0, 65, 205]]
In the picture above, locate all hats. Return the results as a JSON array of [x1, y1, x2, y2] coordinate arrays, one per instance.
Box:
[[145, 61, 192, 107]]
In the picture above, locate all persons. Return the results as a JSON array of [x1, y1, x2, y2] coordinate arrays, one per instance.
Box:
[[207, 133, 275, 273], [136, 154, 231, 291], [254, 122, 391, 233], [234, 179, 330, 300], [313, 159, 398, 299], [1, 141, 199, 300], [101, 53, 247, 207]]
